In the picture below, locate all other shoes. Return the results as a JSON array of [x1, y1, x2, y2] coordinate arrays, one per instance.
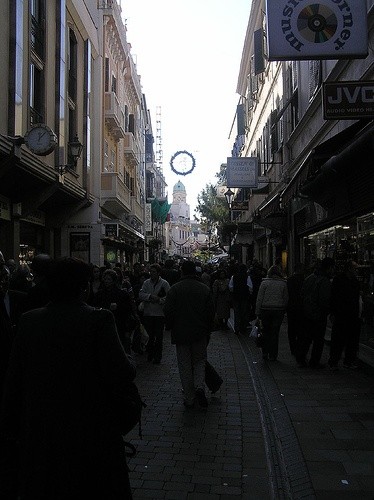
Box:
[[183, 388, 208, 410], [306, 362, 328, 370], [328, 359, 360, 370]]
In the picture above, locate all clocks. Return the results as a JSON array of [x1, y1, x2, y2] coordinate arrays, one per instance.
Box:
[[24, 124, 58, 157]]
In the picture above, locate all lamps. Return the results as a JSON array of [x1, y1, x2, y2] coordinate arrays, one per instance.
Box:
[[55, 132, 82, 177]]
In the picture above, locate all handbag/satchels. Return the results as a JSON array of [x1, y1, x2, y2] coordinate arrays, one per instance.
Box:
[[204, 360, 224, 396], [132, 325, 150, 354], [248, 319, 263, 346]]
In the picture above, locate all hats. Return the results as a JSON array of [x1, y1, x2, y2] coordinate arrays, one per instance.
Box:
[[268, 266, 280, 275]]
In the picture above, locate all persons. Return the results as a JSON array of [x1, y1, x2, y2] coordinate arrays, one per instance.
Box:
[[0, 252, 263, 367], [164, 260, 215, 410], [255, 257, 361, 370], [0, 256, 137, 500]]
